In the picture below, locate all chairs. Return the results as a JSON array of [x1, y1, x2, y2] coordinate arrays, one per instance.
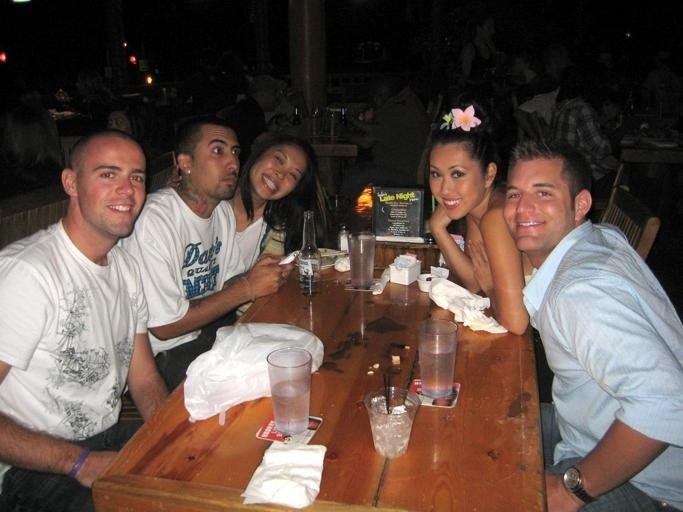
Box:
[[598, 182, 662, 261]]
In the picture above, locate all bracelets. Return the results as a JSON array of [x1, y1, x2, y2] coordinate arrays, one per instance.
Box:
[[65, 443, 93, 479], [237, 273, 258, 305]]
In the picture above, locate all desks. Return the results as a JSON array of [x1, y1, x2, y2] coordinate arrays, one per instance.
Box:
[[312, 144, 359, 197], [610, 124, 683, 229], [91, 244, 548, 512]]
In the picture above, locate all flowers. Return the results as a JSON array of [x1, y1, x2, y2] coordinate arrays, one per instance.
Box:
[[440, 105, 482, 132]]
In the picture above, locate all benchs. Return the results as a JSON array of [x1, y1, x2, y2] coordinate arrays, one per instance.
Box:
[[0, 158, 175, 422]]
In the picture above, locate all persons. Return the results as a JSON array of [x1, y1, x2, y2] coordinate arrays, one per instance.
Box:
[[115, 114, 295, 393], [227, 131, 318, 286], [425, 102, 537, 337], [0, 125, 176, 511], [0, 34, 683, 193], [502, 129, 681, 511]]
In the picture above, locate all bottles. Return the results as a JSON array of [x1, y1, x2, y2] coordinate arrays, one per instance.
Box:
[[337, 224, 348, 252], [297, 211, 323, 294], [291, 104, 349, 136]]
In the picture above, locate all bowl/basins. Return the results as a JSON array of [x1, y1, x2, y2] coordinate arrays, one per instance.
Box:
[[416, 274, 439, 292]]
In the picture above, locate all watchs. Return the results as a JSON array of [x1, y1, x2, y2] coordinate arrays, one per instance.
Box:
[[558, 461, 595, 501]]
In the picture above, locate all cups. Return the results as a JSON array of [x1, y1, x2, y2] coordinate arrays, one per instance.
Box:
[[347, 232, 375, 288], [364, 387, 420, 458], [265, 348, 312, 433], [417, 320, 457, 398]]
